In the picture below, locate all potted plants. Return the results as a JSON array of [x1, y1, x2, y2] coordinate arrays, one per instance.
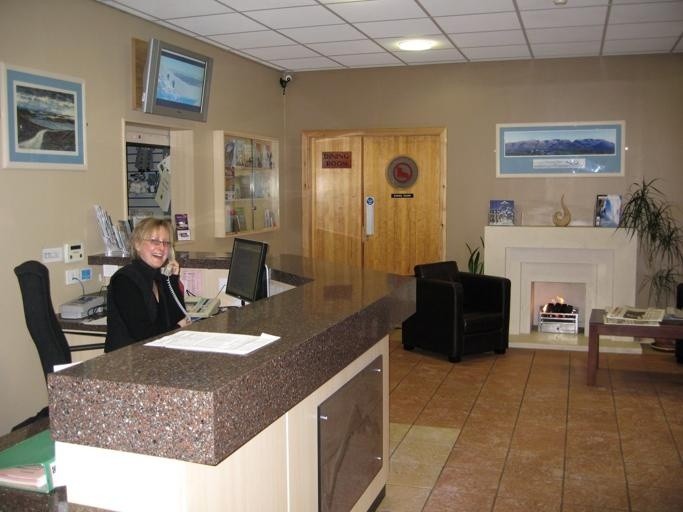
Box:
[[616, 173, 683, 351]]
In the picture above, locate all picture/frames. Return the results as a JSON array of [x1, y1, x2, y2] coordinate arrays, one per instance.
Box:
[[0, 63, 89, 172], [494, 120, 627, 178]]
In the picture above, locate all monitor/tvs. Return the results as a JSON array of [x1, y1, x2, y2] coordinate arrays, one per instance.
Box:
[[225, 237, 268, 302], [143, 37, 214, 123]]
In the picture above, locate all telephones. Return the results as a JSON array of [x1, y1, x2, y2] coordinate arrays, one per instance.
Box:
[[163, 246, 175, 277]]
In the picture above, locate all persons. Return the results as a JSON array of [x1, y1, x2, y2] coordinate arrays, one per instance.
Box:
[[103, 216, 187, 353], [624, 311, 644, 320]]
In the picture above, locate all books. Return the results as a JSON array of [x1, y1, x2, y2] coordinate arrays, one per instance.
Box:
[[92, 204, 132, 251]]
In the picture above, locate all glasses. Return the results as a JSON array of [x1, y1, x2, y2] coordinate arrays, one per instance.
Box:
[[142, 239, 172, 246]]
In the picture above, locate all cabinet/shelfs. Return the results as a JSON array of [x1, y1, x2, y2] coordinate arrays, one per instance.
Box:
[[215, 130, 280, 238]]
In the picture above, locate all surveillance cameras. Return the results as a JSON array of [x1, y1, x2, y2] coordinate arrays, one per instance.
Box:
[[282, 70, 294, 82]]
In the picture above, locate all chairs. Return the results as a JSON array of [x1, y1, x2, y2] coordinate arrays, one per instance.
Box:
[[401, 261, 510, 360], [9, 259, 105, 382]]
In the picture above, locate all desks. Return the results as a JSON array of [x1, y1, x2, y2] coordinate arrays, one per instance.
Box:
[[585, 307, 683, 372]]
